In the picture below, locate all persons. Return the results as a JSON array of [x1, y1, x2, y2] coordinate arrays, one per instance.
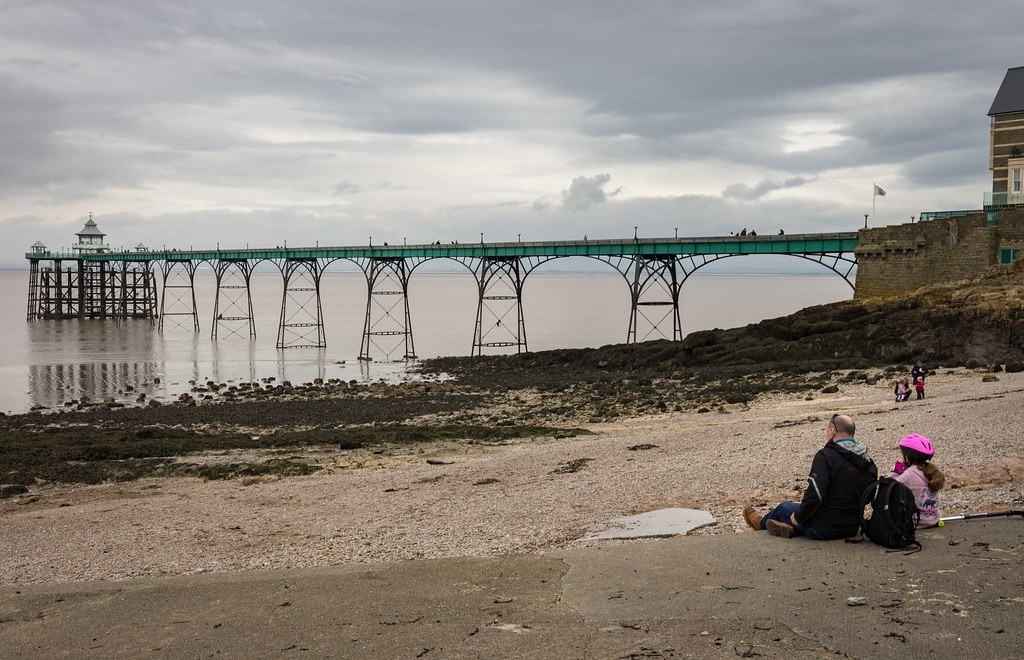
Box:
[[895, 361, 929, 402], [728, 228, 757, 236], [885, 433, 945, 529], [778, 229, 784, 235], [743, 415, 878, 541]]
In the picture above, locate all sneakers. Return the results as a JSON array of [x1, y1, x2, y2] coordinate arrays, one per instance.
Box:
[[766, 519, 797, 538], [743, 507, 763, 531]]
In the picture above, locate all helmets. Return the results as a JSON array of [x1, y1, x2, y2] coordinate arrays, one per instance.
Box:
[[899, 434, 935, 461]]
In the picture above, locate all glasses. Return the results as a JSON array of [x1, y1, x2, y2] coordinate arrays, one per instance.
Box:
[[831, 414, 840, 433]]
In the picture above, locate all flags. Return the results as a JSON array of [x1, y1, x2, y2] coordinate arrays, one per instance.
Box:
[[875, 186, 886, 197]]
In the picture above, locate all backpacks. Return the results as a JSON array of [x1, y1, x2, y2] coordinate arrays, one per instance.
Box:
[[845, 476, 922, 555]]
